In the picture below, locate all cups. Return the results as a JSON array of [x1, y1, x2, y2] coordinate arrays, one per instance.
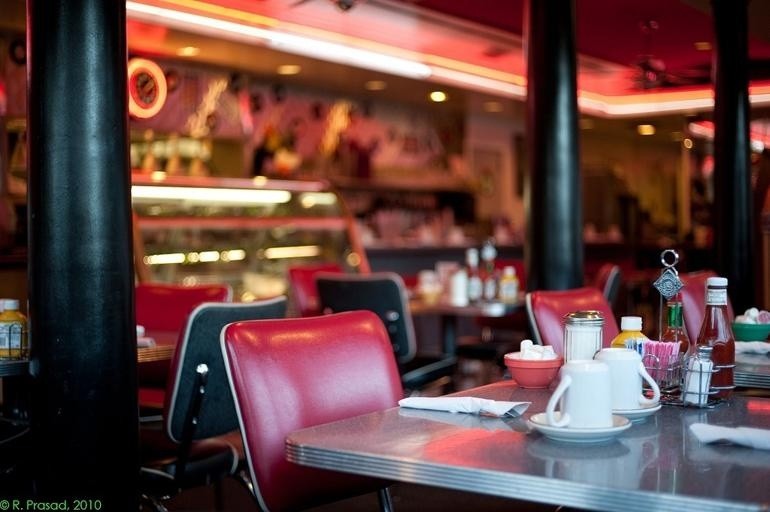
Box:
[[543, 457, 614, 489], [546, 360, 613, 428], [593, 348, 661, 409], [616, 439, 658, 490]]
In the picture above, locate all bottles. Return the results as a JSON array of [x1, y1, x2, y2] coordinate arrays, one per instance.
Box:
[[697, 276, 735, 397], [662, 426, 682, 496], [681, 413, 713, 481], [450, 236, 518, 310], [610, 313, 653, 348], [681, 344, 714, 405], [561, 308, 605, 360], [1, 299, 30, 359], [663, 301, 692, 354], [706, 429, 737, 508]]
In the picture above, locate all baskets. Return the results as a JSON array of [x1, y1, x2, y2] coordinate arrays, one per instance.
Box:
[[642, 249, 737, 409], [0, 299, 31, 361]]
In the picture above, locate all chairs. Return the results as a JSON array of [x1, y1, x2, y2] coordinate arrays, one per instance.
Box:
[[675, 270, 736, 348], [221, 308, 405, 511], [136, 279, 232, 424], [141, 295, 291, 511], [290, 257, 620, 390]]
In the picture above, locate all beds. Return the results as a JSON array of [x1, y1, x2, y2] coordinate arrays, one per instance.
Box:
[[1, 333, 183, 445]]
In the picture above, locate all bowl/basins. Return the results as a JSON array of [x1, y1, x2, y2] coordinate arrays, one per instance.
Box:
[[503, 350, 561, 388], [733, 321, 770, 342]]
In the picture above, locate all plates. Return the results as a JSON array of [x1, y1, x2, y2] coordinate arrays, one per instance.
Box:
[[615, 404, 666, 423], [526, 436, 630, 463], [529, 410, 633, 443], [619, 418, 662, 442]]
